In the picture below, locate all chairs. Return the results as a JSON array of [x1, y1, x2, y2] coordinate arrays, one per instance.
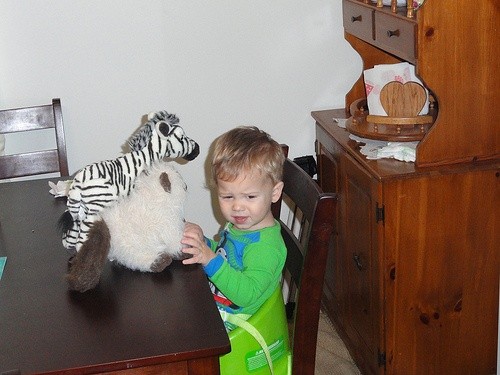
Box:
[[0, 98, 69, 180], [272, 143, 338, 375]]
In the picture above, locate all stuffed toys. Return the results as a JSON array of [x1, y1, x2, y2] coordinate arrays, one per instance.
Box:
[[68, 159, 192, 294], [57, 112, 200, 253]]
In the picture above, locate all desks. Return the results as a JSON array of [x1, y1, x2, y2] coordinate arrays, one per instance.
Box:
[[0, 174, 232, 375]]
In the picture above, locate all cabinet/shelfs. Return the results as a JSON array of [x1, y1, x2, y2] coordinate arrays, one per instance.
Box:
[[311, 0, 500, 375]]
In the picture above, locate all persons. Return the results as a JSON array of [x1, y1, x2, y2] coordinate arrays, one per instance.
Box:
[[181, 126, 287, 337]]
